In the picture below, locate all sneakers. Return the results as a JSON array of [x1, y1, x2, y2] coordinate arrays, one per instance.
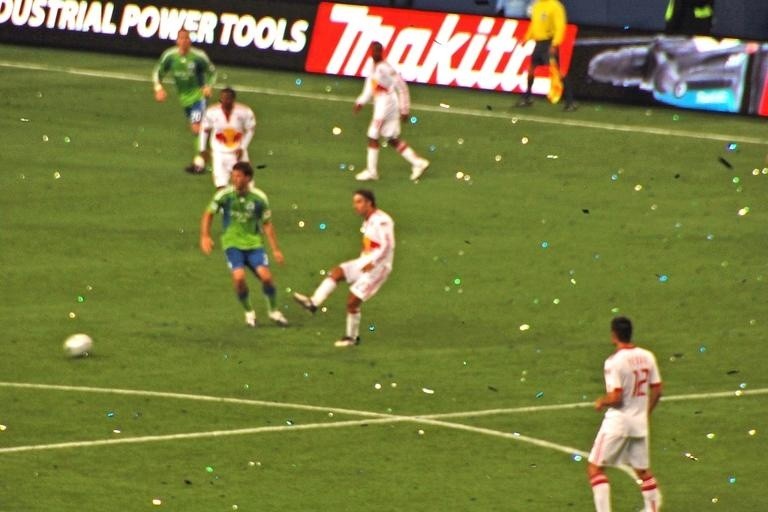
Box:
[[293, 292, 317, 313], [356, 170, 379, 181], [410, 159, 430, 180], [244, 310, 257, 327], [334, 335, 361, 348], [269, 308, 288, 326]]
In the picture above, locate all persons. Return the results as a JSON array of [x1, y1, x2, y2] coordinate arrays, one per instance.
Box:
[[513, 0, 581, 113], [152, 28, 218, 174], [352, 41, 429, 183], [192, 88, 257, 215], [292, 189, 397, 347], [586, 315, 665, 512], [199, 162, 291, 328]]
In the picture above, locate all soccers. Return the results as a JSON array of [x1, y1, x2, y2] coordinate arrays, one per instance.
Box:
[[64, 333, 92, 358]]
